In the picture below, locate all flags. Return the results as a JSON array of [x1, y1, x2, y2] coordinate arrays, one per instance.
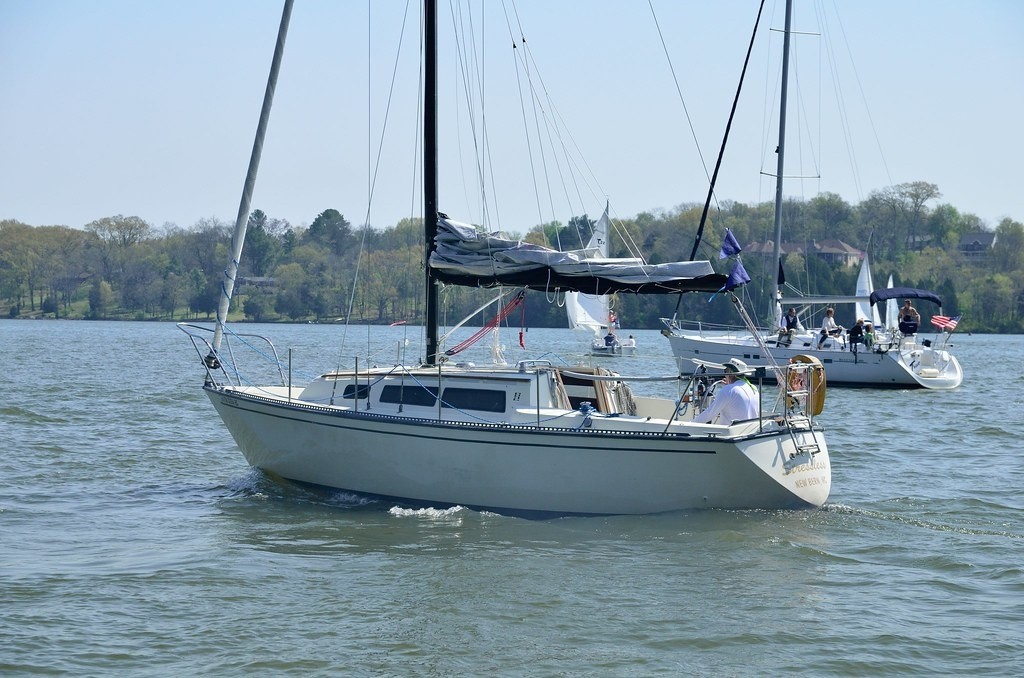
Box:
[[930, 311, 964, 331]]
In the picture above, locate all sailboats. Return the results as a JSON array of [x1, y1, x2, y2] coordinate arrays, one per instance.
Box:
[[662, 0, 967, 394], [564, 200, 641, 360], [178, 2, 848, 519]]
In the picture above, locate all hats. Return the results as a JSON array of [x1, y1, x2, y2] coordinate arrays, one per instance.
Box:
[[722, 357, 752, 376]]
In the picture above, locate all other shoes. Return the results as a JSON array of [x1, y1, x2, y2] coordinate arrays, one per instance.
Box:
[[834, 334, 839, 339], [816, 344, 822, 350]]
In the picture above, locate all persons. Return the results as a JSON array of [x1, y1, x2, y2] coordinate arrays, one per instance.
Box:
[[629, 335, 635, 346], [689, 357, 759, 425], [898, 299, 920, 326], [849, 319, 878, 352], [604, 333, 617, 354], [776, 308, 804, 348], [817, 307, 842, 350]]
